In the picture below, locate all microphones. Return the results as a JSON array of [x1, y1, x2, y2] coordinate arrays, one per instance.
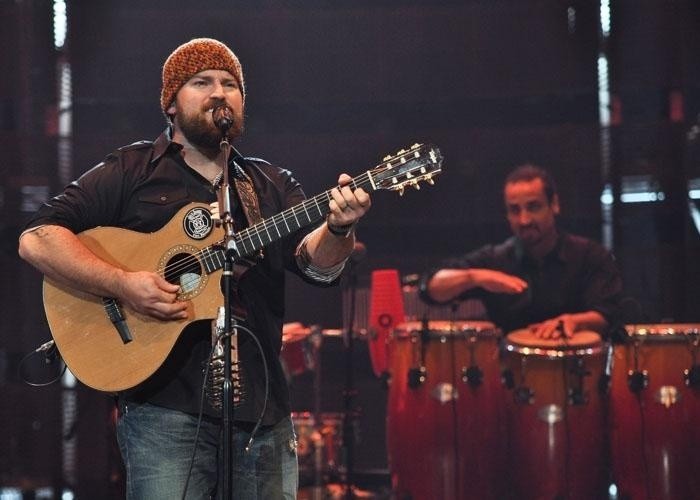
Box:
[[211, 100, 235, 128]]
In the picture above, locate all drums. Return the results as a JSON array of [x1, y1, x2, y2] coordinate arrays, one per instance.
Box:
[[386, 321, 504, 500], [281, 320, 312, 376], [295, 412, 346, 484], [505, 327, 609, 500], [610, 324, 700, 500]]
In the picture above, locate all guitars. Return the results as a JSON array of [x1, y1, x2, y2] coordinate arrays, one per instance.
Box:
[[42, 142, 444, 393]]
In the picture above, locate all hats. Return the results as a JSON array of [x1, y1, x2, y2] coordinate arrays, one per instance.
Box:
[[159, 37, 246, 119]]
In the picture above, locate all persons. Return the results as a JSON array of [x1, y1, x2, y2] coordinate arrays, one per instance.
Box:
[[7, 32, 371, 500], [419, 162, 627, 345]]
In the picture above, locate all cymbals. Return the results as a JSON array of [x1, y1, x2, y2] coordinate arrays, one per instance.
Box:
[[322, 327, 367, 339]]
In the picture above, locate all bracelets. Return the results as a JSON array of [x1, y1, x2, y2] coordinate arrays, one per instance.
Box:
[[327, 225, 355, 236]]
